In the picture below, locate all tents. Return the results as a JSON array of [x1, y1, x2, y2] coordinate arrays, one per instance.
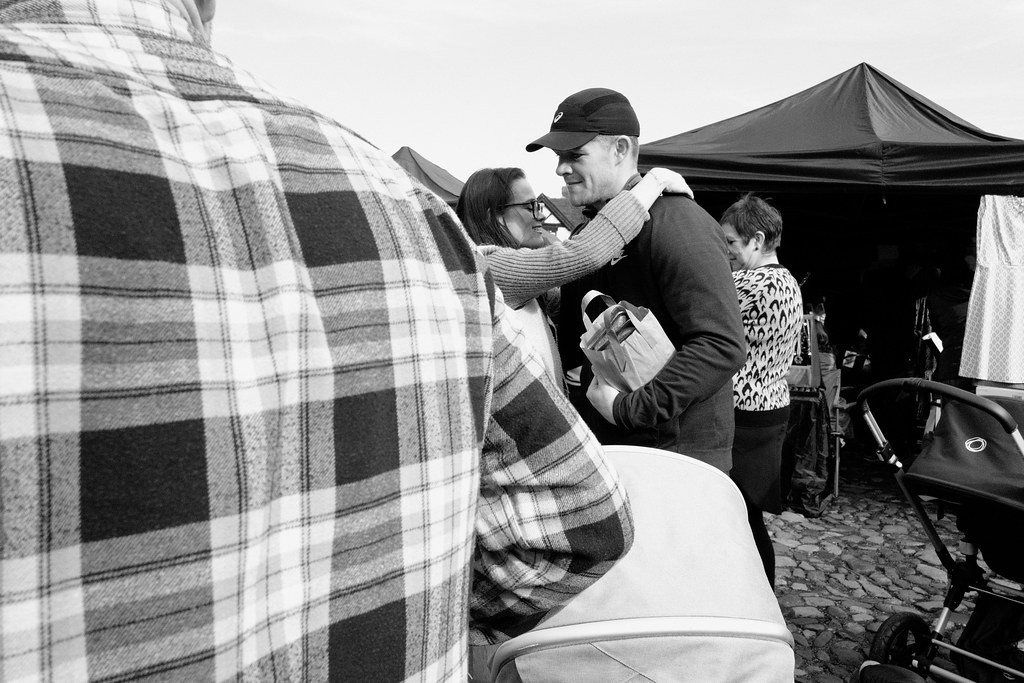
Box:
[[639, 61, 1024, 192], [391, 147, 465, 211]]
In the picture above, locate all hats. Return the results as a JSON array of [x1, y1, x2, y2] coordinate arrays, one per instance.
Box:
[[525, 88, 640, 152]]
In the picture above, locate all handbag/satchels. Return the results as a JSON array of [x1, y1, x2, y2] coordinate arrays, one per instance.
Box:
[[580, 290, 677, 393]]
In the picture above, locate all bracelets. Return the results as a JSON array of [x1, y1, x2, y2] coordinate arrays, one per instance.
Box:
[[647, 170, 666, 196]]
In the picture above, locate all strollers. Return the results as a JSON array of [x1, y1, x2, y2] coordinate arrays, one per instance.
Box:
[[486, 446, 796, 683], [855, 375, 1024, 683]]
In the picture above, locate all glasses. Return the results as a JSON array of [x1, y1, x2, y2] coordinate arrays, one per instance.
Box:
[[498, 199, 543, 221]]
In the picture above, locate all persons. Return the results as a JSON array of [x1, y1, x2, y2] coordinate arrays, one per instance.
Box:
[[0, 0, 634, 683], [718, 193, 804, 592], [453, 87, 747, 478]]
